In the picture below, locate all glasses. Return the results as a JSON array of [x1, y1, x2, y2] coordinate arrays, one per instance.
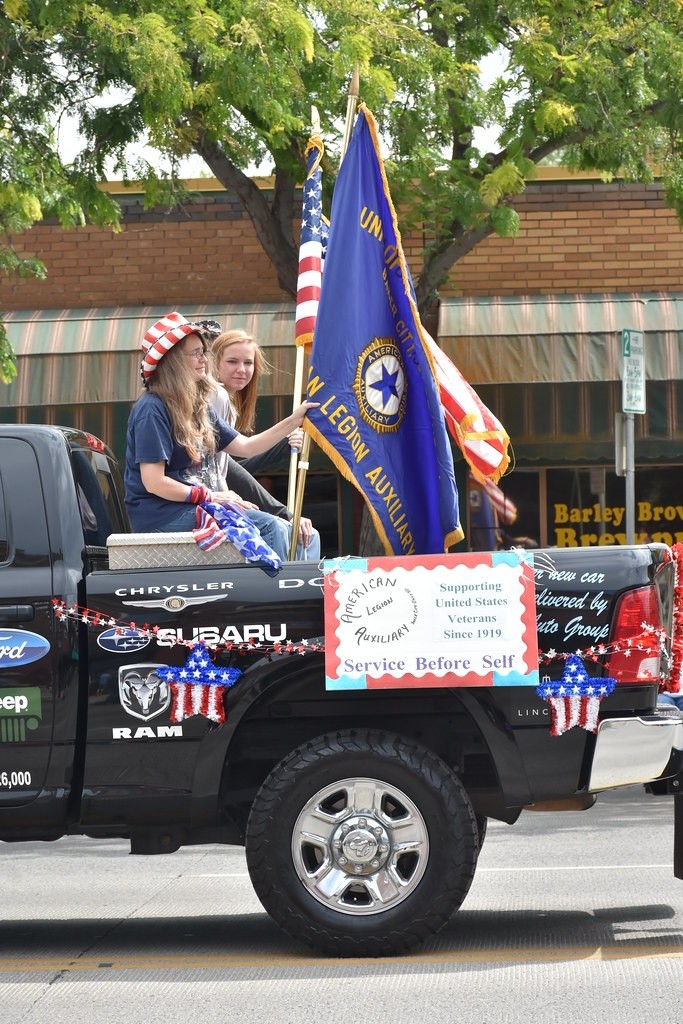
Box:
[[180, 350, 205, 359]]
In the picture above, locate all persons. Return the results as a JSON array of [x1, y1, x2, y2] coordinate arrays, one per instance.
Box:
[[122, 311, 321, 563], [186, 328, 320, 561]]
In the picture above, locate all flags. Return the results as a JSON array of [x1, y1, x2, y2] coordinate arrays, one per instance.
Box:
[[468, 470, 517, 525], [293, 133, 511, 483], [302, 103, 466, 556], [467, 483, 501, 551]]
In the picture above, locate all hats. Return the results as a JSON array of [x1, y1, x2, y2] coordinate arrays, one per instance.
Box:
[[139, 312, 221, 388]]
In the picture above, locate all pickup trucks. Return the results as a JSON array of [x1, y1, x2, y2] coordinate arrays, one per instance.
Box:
[[0, 422, 682, 958]]
